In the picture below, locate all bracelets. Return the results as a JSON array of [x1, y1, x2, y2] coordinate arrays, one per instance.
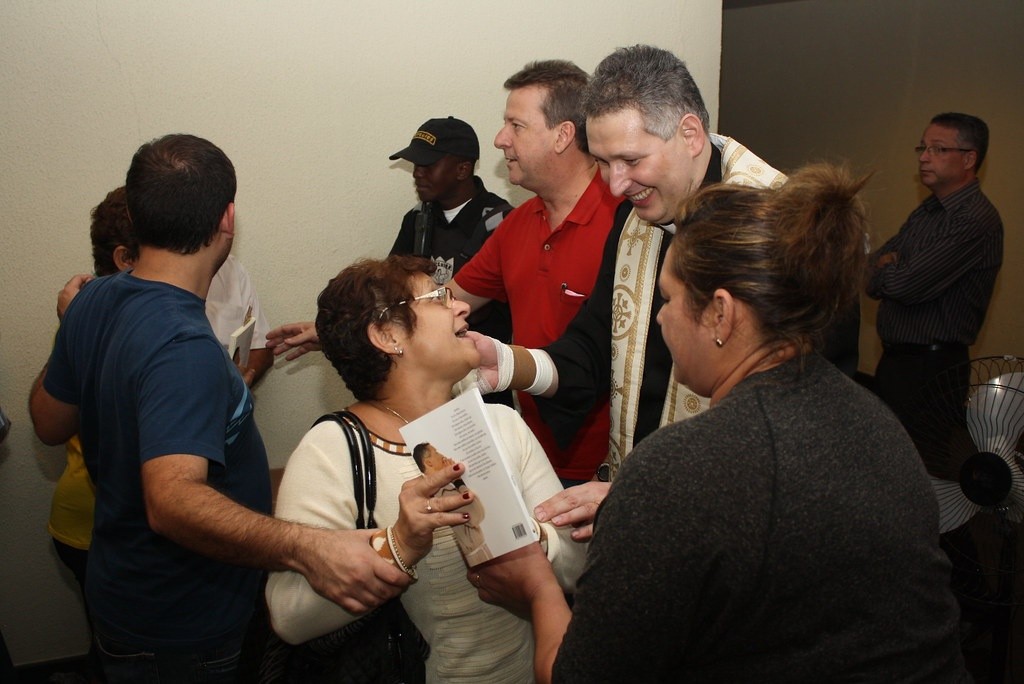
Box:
[[390, 529, 415, 576]]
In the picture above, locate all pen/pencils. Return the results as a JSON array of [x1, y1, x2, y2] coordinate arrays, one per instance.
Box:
[[559, 282, 567, 300]]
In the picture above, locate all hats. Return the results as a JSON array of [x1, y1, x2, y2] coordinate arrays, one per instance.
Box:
[[389, 116, 479, 165]]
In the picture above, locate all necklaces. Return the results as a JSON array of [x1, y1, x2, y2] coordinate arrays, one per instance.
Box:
[[379, 401, 408, 424]]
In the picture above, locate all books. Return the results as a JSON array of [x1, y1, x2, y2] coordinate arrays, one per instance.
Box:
[[400, 388, 539, 567], [228, 316, 256, 375]]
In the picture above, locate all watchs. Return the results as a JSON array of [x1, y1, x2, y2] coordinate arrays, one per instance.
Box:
[[596, 462, 609, 482]]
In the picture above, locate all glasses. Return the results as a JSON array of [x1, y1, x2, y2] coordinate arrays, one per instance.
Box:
[[915, 146, 972, 155], [377, 287, 456, 320]]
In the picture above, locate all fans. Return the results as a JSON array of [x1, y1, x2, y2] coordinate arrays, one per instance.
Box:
[[919, 354, 1024, 684]]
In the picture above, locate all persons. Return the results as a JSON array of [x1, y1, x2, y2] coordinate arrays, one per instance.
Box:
[[466, 44, 789, 541], [865, 111, 1005, 474], [30, 134, 408, 684], [204, 253, 274, 389], [265, 58, 625, 490], [265, 254, 589, 684], [469, 165, 970, 684], [232, 346, 241, 366], [412, 442, 494, 567], [389, 116, 515, 409], [49, 186, 139, 585]]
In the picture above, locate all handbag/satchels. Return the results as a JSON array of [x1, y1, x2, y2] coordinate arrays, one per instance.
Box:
[[259, 412, 428, 684]]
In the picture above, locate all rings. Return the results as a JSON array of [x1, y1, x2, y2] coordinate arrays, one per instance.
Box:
[[427, 500, 431, 513], [476, 575, 480, 587]]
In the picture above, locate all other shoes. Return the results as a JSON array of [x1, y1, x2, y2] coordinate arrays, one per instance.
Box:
[[958, 593, 994, 647]]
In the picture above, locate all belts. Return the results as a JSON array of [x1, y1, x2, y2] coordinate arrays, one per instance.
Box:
[[881, 342, 957, 353]]
